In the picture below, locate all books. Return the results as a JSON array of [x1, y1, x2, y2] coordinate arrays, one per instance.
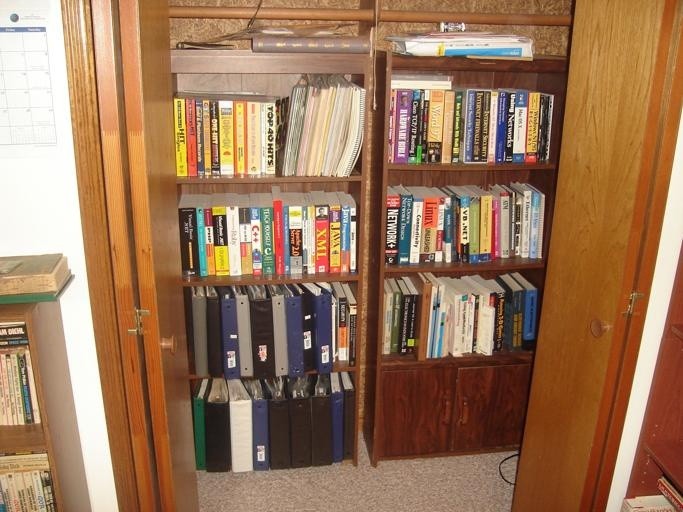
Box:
[[326, 270, 539, 368], [173, 72, 366, 278], [0, 252, 76, 512], [620, 476, 683, 512], [252, 33, 372, 54], [390, 31, 555, 268]]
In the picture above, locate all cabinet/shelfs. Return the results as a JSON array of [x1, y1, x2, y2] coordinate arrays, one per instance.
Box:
[[166, 45, 376, 474], [372, 45, 570, 358], [616, 241, 681, 512], [362, 350, 533, 469], [0, 275, 91, 512]]
